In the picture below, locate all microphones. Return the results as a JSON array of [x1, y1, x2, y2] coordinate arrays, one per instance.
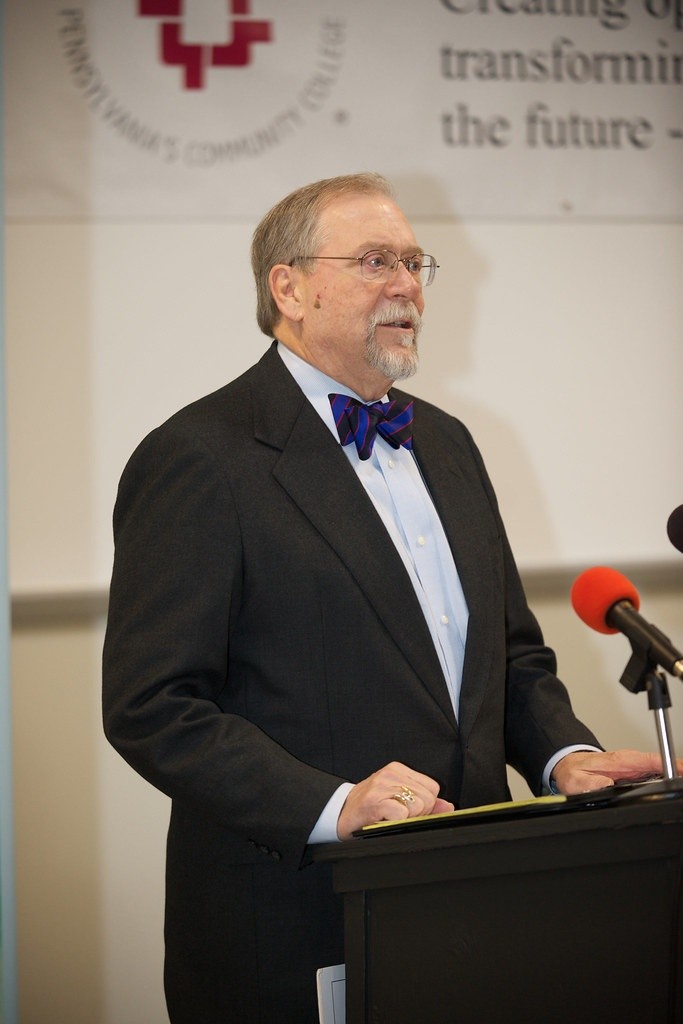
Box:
[[666, 504, 683, 553], [570, 567, 683, 683]]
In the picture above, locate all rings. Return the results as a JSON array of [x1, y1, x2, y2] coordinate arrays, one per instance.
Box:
[[393, 786, 416, 806]]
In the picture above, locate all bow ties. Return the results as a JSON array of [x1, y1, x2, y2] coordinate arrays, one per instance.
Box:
[[328, 393, 414, 460]]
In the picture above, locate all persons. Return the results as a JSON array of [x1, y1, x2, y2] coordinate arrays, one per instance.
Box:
[[97, 176, 683, 1024]]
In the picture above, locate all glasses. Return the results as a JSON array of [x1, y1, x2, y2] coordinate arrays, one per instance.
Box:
[[288, 250, 440, 287]]
